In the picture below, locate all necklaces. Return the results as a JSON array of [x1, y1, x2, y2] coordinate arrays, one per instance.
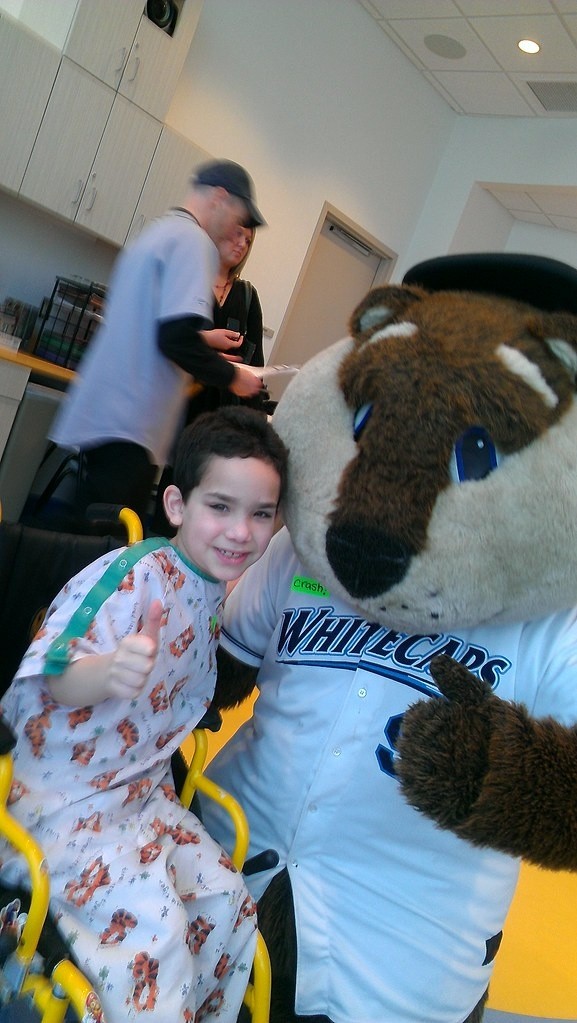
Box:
[[215, 277, 227, 304]]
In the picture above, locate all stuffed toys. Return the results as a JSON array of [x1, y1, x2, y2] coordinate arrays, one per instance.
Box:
[[189, 254, 577, 1023]]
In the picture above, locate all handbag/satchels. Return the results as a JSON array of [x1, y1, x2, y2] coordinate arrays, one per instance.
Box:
[[239, 280, 257, 365]]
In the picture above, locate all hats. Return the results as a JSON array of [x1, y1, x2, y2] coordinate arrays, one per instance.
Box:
[[191, 158, 269, 228]]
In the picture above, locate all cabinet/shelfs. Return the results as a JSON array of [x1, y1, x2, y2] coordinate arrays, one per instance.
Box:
[[0, 0, 219, 252]]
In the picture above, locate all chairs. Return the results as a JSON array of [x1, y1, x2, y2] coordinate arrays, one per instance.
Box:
[[0, 500, 273, 1023]]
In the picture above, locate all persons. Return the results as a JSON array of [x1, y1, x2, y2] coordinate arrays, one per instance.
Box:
[[154, 229, 269, 527], [48, 158, 268, 598], [0, 409, 289, 1023]]
